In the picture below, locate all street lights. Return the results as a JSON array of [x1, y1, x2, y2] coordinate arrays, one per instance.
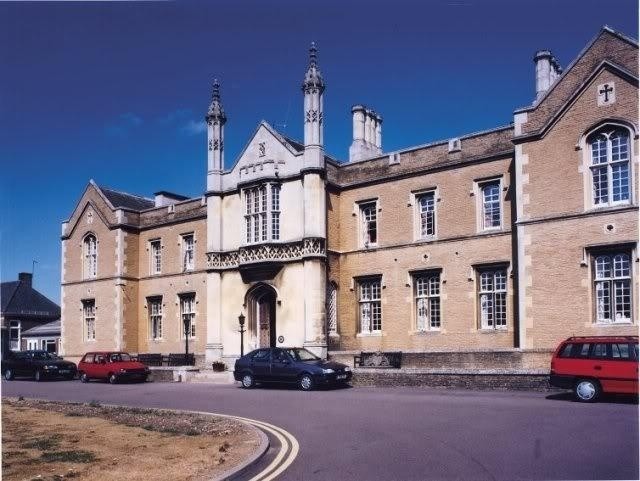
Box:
[[184, 317, 191, 355], [238, 313, 247, 359]]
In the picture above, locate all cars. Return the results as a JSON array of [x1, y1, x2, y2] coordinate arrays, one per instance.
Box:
[[234, 346, 352, 391], [549, 334, 639, 402], [2, 350, 76, 382]]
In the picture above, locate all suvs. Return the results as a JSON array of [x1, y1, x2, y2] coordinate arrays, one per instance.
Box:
[[78, 351, 151, 384]]
